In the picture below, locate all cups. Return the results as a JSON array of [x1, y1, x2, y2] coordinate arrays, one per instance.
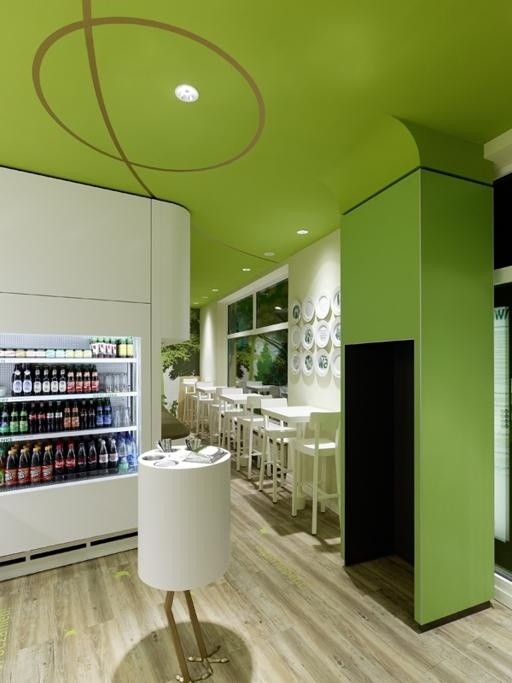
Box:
[[159, 439, 173, 453], [184, 439, 202, 453]]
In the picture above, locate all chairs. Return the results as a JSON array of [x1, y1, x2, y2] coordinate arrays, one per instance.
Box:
[[294, 411, 342, 534], [261, 398, 295, 503], [179, 376, 271, 479]]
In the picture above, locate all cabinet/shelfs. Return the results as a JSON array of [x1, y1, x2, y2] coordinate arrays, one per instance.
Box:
[[1, 331, 141, 583]]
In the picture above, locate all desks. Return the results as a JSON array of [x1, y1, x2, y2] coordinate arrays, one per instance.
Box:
[[263, 404, 332, 509], [219, 392, 262, 418], [197, 385, 227, 415]]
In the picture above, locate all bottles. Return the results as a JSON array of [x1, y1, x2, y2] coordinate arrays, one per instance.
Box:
[[0, 450, 5, 486], [117, 434, 128, 473], [25, 448, 31, 463], [89, 364, 93, 379], [91, 365, 99, 392], [90, 337, 99, 357], [98, 336, 105, 357], [34, 444, 42, 456], [88, 398, 96, 427], [19, 403, 28, 433], [80, 399, 88, 429], [55, 401, 63, 432], [116, 340, 119, 356], [23, 363, 32, 396], [64, 399, 71, 430], [0, 403, 3, 421], [11, 447, 18, 463], [126, 337, 133, 357], [23, 446, 30, 460], [42, 366, 50, 394], [30, 447, 41, 484], [89, 435, 97, 475], [3, 442, 8, 460], [72, 399, 80, 430], [84, 365, 91, 393], [58, 365, 66, 393], [10, 403, 18, 434], [118, 338, 126, 357], [109, 433, 118, 473], [46, 400, 54, 432], [5, 450, 17, 486], [51, 365, 58, 394], [0, 402, 9, 436], [95, 398, 103, 427], [65, 438, 76, 478], [28, 402, 37, 433], [33, 365, 41, 394], [26, 442, 32, 453], [103, 397, 112, 426], [126, 431, 136, 472], [81, 364, 85, 381], [99, 435, 108, 475], [55, 438, 65, 481], [76, 366, 83, 393], [78, 436, 86, 479], [67, 365, 75, 393], [111, 337, 116, 357], [37, 401, 46, 432], [12, 364, 22, 396], [104, 338, 111, 357], [18, 449, 30, 485], [42, 438, 54, 482], [14, 444, 19, 451]]
